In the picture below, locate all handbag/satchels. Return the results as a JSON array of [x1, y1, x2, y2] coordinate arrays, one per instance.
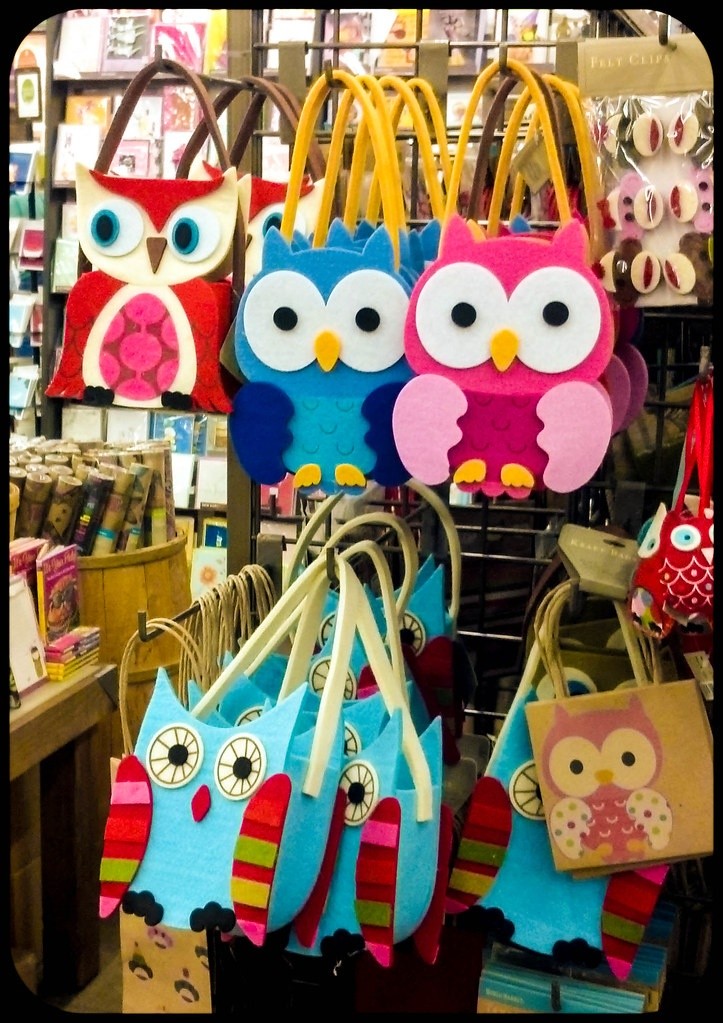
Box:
[[41, 57, 714, 984]]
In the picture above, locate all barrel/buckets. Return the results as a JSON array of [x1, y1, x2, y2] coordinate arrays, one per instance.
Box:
[[76, 527, 192, 864]]
